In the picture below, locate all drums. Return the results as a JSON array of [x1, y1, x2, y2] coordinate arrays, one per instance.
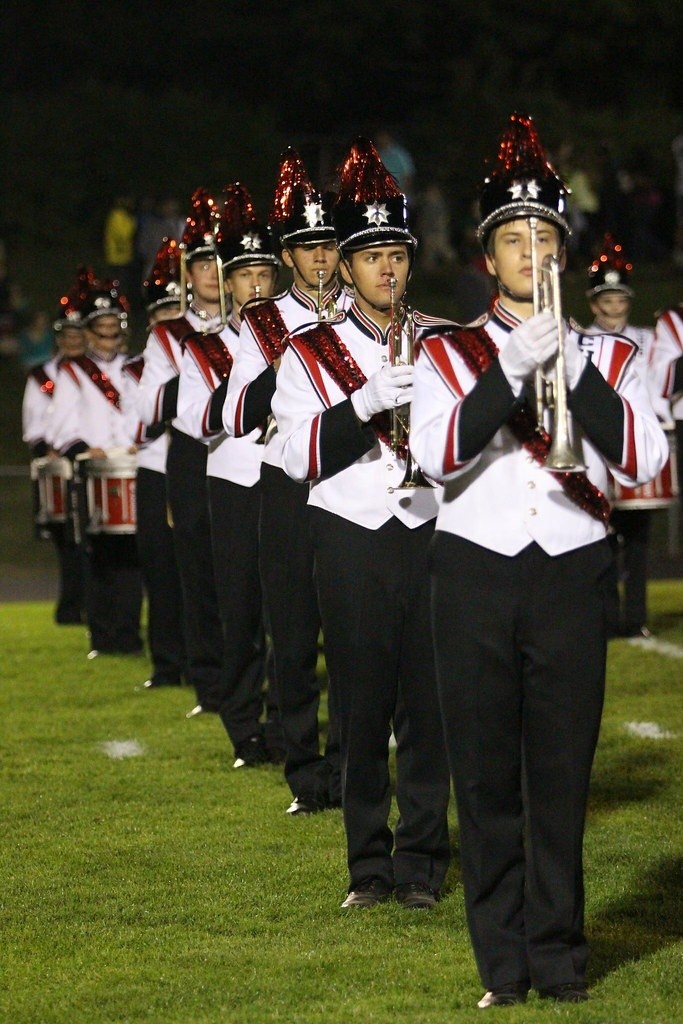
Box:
[[31, 455, 73, 525], [84, 459, 138, 534], [607, 431, 680, 510]]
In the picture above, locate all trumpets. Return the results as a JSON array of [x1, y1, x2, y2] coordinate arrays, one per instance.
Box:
[[316, 270, 338, 323], [528, 214, 590, 475], [387, 276, 437, 493], [254, 285, 261, 305]]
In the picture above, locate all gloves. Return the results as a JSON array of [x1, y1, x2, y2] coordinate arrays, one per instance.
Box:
[[497, 308, 559, 397], [547, 318, 587, 391], [350, 362, 413, 422]]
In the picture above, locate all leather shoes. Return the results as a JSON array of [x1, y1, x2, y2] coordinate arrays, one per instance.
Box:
[[233, 742, 260, 767], [285, 796, 313, 814], [136, 680, 159, 694], [394, 883, 440, 909], [541, 981, 590, 1003], [477, 991, 527, 1007], [342, 879, 389, 906], [185, 703, 218, 720]]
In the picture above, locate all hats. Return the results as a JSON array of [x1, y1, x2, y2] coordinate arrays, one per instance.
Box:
[[217, 182, 281, 269], [477, 112, 573, 241], [53, 283, 88, 330], [142, 237, 181, 316], [183, 187, 219, 262], [334, 135, 413, 251], [586, 234, 635, 297], [268, 145, 336, 243], [79, 270, 127, 325]]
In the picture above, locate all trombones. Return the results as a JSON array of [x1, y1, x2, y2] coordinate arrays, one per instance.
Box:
[[213, 220, 228, 326], [146, 217, 189, 331]]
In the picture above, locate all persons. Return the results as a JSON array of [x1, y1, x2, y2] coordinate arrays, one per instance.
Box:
[[584, 237, 682, 636], [408, 110, 670, 1007], [277, 138, 452, 909], [24, 156, 343, 819]]
